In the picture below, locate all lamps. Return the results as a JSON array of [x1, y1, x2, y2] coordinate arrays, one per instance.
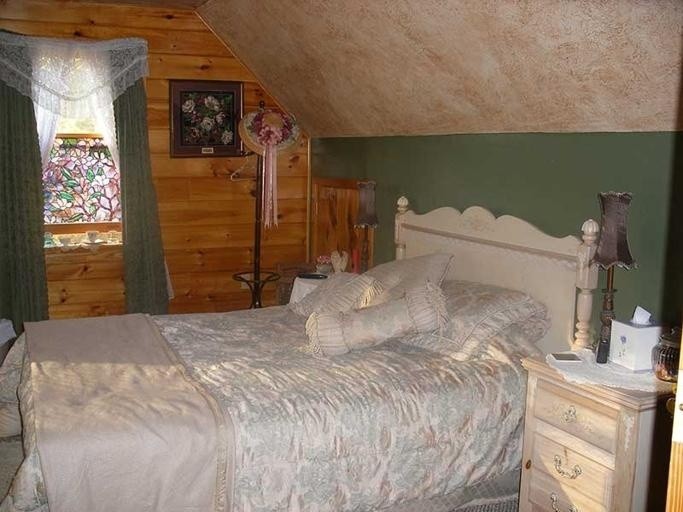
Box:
[[358, 180, 379, 273], [590, 191, 638, 363]]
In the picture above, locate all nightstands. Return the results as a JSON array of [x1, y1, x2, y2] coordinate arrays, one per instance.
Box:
[[517, 353, 675, 512]]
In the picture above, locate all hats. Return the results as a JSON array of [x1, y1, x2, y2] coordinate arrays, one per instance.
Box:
[[238, 108, 301, 156]]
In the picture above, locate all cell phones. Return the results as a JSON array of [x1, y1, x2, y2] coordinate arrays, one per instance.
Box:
[[549, 352, 582, 363]]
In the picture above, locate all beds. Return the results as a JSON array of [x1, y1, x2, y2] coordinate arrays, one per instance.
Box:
[[0, 196, 601, 512]]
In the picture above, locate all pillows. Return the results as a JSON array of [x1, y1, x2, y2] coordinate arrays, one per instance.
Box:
[[288, 253, 546, 364]]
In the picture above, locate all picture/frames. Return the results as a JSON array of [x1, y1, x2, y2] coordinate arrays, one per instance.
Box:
[[168, 79, 246, 157]]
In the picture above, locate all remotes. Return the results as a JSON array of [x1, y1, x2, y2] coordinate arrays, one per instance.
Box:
[[300, 273, 326, 278]]
